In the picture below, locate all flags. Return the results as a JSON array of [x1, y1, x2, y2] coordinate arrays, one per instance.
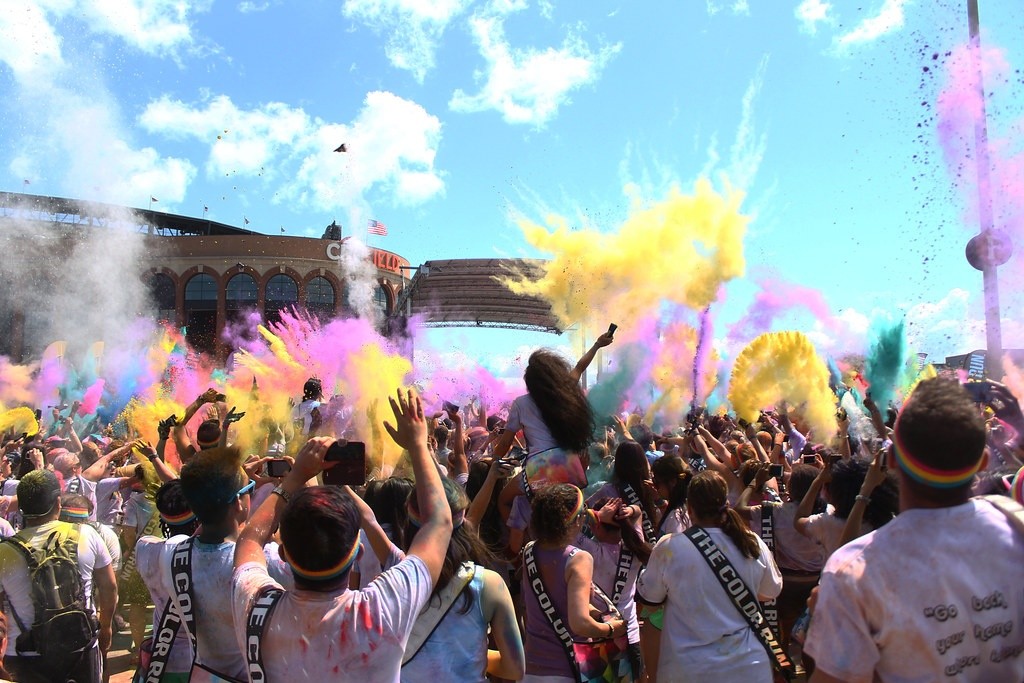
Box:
[[24, 180, 30, 184], [205, 207, 208, 211], [245, 217, 249, 224], [281, 228, 286, 232], [367, 219, 387, 237], [152, 197, 158, 202]]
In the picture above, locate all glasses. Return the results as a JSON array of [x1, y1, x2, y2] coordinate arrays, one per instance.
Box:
[[227, 479, 256, 504], [2, 457, 10, 464], [584, 503, 588, 510]]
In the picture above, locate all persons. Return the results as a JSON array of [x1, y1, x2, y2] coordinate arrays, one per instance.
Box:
[[0, 377, 1024, 683], [486, 332, 599, 559]]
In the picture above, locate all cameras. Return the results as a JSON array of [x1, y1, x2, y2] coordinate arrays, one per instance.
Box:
[[25, 451, 31, 459]]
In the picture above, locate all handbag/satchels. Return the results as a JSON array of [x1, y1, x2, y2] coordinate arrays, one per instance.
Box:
[[567, 633, 634, 683], [132, 636, 153, 683]]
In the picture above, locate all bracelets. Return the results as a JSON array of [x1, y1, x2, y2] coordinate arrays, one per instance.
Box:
[[855, 495, 870, 505], [606, 622, 614, 638], [223, 422, 230, 429], [271, 486, 291, 504], [587, 509, 599, 525], [774, 443, 783, 447], [160, 436, 169, 440], [746, 484, 757, 490], [693, 433, 700, 438], [839, 435, 849, 438], [594, 342, 601, 348], [770, 424, 774, 428], [147, 452, 159, 462], [629, 506, 635, 516], [198, 394, 207, 404], [748, 436, 756, 440]]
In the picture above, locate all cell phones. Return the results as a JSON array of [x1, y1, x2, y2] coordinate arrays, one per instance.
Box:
[[322, 441, 366, 486], [879, 450, 888, 467], [802, 455, 818, 464], [497, 428, 506, 435], [862, 438, 870, 450], [600, 610, 628, 629], [768, 464, 784, 477], [873, 438, 882, 453], [962, 382, 991, 402], [268, 459, 291, 478], [830, 454, 842, 464]]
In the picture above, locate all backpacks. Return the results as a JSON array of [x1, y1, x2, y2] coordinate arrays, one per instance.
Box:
[[0, 523, 100, 653]]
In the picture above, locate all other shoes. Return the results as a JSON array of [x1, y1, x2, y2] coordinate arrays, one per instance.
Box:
[[114, 616, 130, 630], [129, 647, 140, 665]]
